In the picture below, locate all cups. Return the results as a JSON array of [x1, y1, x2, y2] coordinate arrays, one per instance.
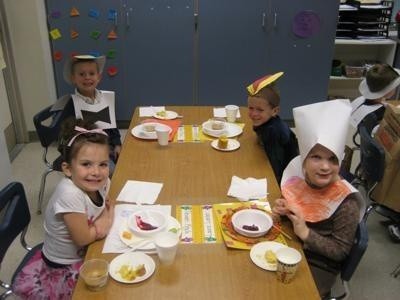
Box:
[[80, 258, 109, 293], [155, 126, 171, 146], [153, 230, 178, 265], [225, 105, 239, 122], [276, 247, 302, 284]]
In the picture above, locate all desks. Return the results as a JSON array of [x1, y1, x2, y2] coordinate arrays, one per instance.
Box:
[[71, 104, 322, 300]]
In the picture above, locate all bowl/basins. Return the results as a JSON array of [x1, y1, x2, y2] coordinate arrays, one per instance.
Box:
[[139, 123, 157, 138], [202, 121, 229, 135], [127, 209, 167, 234], [231, 209, 275, 237]]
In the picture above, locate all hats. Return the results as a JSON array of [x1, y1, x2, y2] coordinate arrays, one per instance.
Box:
[[292, 98, 352, 168], [63, 55, 107, 84], [359, 68, 400, 99]]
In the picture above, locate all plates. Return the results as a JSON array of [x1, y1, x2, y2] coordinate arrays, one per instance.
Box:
[[108, 251, 157, 285], [154, 111, 178, 121], [210, 139, 241, 152], [131, 122, 172, 141], [222, 206, 284, 244], [249, 240, 290, 272], [206, 123, 244, 138], [120, 216, 182, 252]]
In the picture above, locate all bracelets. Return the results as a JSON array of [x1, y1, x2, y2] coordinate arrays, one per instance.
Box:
[[93, 224, 102, 240]]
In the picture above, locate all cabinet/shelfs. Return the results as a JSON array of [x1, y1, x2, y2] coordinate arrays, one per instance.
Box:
[[195, 1, 340, 120], [45, 0, 195, 126], [326, 36, 398, 101]]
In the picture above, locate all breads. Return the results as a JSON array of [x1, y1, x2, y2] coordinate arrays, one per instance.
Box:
[[217, 135, 228, 149], [119, 264, 146, 281], [265, 249, 275, 263]]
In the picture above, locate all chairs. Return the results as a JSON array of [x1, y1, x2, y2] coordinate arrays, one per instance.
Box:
[[331, 219, 368, 300], [31, 104, 117, 216], [348, 118, 400, 278], [1, 182, 44, 300]]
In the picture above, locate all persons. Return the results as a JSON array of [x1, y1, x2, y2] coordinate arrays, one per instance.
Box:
[[248, 73, 300, 187], [273, 100, 367, 298], [41, 120, 114, 271], [51, 55, 122, 179], [344, 63, 400, 148]]
